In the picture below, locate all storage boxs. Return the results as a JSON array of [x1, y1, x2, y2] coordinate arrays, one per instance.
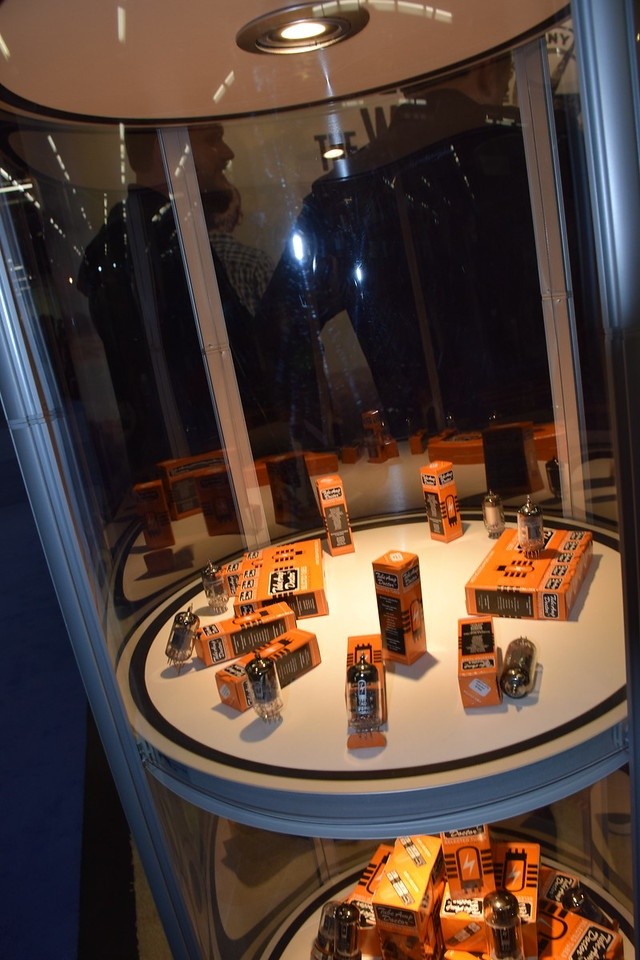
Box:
[[345, 826, 625, 960], [194, 473, 595, 727], [108, 409, 576, 573]]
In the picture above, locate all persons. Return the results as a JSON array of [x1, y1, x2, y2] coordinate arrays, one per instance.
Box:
[[76, 120, 340, 470], [253, 52, 602, 488]]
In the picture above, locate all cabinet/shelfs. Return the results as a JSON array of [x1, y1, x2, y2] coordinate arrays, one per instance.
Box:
[[0, 0, 640, 960]]
